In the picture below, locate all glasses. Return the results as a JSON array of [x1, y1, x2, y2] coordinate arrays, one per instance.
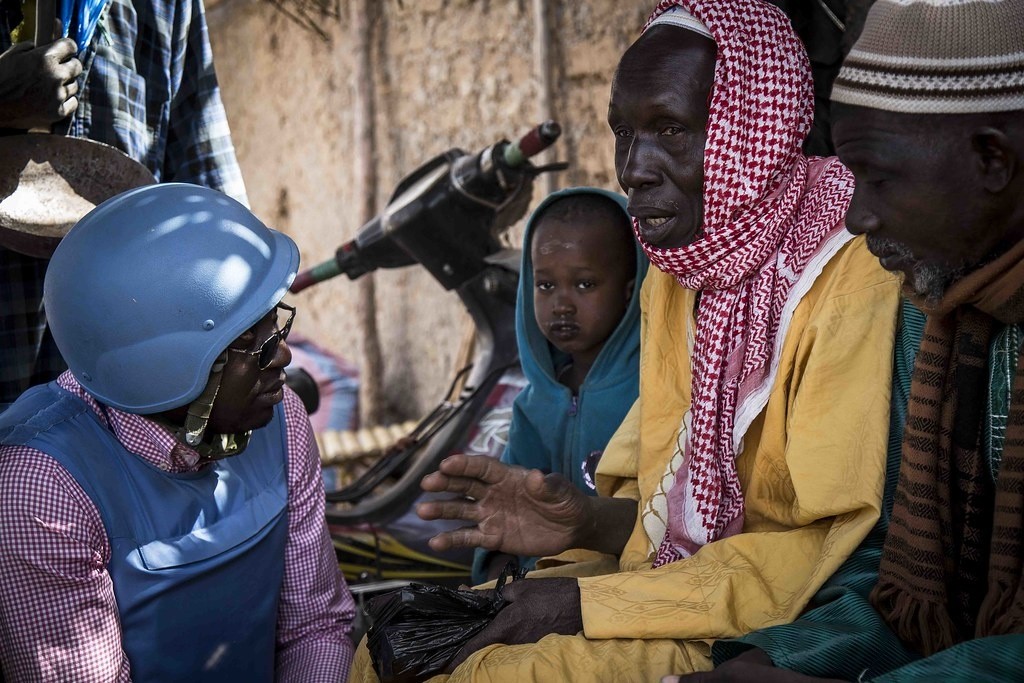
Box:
[[229, 301, 296, 370]]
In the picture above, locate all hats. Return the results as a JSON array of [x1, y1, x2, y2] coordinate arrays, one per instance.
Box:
[[641, 4, 716, 39], [830, 0, 1024, 113]]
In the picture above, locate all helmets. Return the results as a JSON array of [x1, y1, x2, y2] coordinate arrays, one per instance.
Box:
[[42, 182, 300, 414]]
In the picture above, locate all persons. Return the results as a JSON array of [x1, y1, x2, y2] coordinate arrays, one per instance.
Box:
[[348, 0, 903, 683], [661, 0, 1024, 683], [0, 0, 248, 415], [1, 182, 356, 682]]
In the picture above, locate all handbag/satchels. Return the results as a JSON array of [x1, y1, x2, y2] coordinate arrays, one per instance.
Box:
[[365, 561, 525, 683]]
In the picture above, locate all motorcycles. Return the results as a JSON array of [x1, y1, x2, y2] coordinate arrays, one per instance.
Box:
[[286, 116, 574, 611]]
